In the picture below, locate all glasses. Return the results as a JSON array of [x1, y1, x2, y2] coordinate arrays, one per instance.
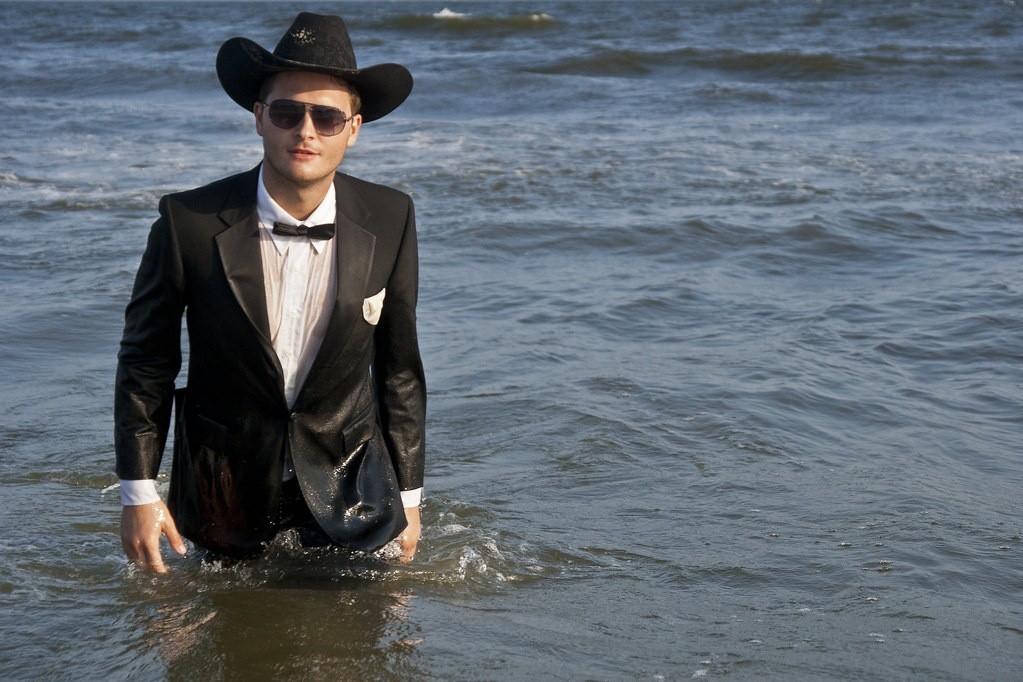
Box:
[[262, 99, 355, 136]]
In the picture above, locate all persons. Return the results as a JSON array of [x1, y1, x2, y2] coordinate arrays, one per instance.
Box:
[[115, 13, 426, 571]]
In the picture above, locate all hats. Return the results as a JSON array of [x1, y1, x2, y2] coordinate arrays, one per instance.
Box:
[[216, 12, 414, 123]]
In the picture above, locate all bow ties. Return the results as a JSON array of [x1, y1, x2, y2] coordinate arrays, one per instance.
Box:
[[272, 221, 335, 240]]
